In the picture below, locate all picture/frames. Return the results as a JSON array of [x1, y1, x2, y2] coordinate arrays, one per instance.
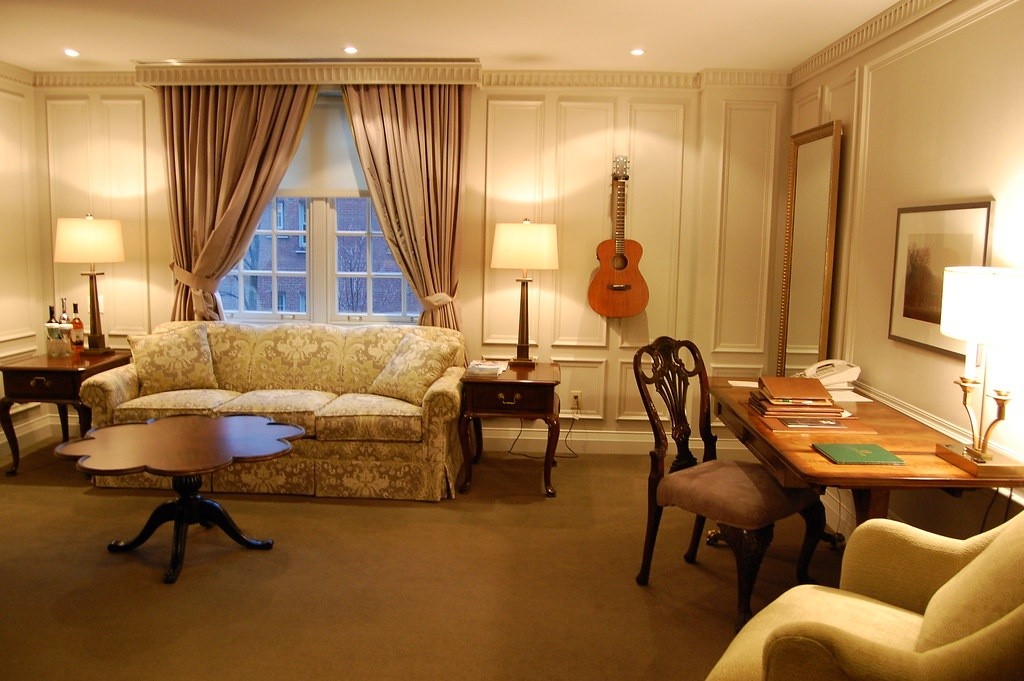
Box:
[[887, 201, 995, 361]]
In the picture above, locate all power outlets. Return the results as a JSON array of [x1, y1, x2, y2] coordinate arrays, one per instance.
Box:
[[570, 391, 582, 411]]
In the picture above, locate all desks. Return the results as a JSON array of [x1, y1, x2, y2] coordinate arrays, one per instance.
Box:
[[0, 350, 132, 478], [454, 359, 561, 498], [707, 370, 1024, 526], [54, 414, 306, 583]]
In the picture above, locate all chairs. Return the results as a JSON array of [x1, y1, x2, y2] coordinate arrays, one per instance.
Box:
[[633, 335, 827, 633]]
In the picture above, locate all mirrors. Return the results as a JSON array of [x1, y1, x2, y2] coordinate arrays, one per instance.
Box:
[[777, 119, 841, 380]]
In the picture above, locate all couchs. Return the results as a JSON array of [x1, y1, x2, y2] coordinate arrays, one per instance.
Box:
[[77, 321, 469, 504], [703, 507, 1023, 681]]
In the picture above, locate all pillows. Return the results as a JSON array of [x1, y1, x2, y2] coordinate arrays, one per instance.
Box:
[[368, 333, 461, 405], [126, 324, 219, 396]]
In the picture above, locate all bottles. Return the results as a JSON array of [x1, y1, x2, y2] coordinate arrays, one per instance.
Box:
[[46, 306, 61, 359], [70, 303, 85, 363], [58, 298, 71, 359]]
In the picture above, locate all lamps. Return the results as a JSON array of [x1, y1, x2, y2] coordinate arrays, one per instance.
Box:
[[934, 266, 1024, 481], [52, 215, 127, 356], [491, 219, 559, 366]]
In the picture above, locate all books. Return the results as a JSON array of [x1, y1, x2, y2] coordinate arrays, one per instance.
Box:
[[811, 444, 905, 465], [747, 375, 860, 428]]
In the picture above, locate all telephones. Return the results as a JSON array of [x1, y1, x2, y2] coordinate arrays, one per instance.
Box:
[[801, 359, 862, 390]]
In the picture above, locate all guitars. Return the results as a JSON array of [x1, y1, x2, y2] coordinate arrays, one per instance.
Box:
[[587, 156, 650, 319]]
